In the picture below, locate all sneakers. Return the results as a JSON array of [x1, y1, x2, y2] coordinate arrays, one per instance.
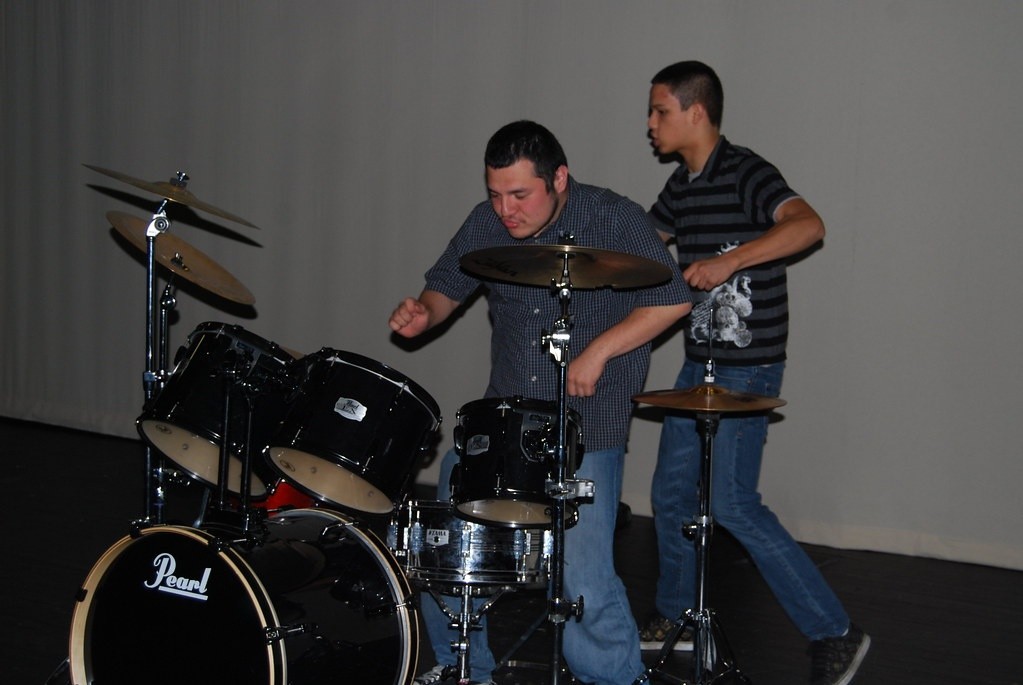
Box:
[[412, 662, 461, 685], [805, 621, 871, 685], [637, 617, 695, 652]]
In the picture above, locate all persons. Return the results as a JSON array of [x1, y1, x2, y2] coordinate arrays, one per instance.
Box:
[[388, 120, 694, 685], [638, 59, 873, 684]]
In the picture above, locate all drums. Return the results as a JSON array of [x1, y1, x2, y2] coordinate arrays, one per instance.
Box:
[[395, 498, 551, 599], [449, 394, 585, 531], [263, 345, 442, 520], [133, 320, 298, 504], [68, 508, 422, 685]]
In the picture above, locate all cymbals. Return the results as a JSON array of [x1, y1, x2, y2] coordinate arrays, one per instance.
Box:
[[630, 383, 788, 413], [78, 160, 266, 233], [104, 209, 258, 307], [456, 241, 676, 293]]
